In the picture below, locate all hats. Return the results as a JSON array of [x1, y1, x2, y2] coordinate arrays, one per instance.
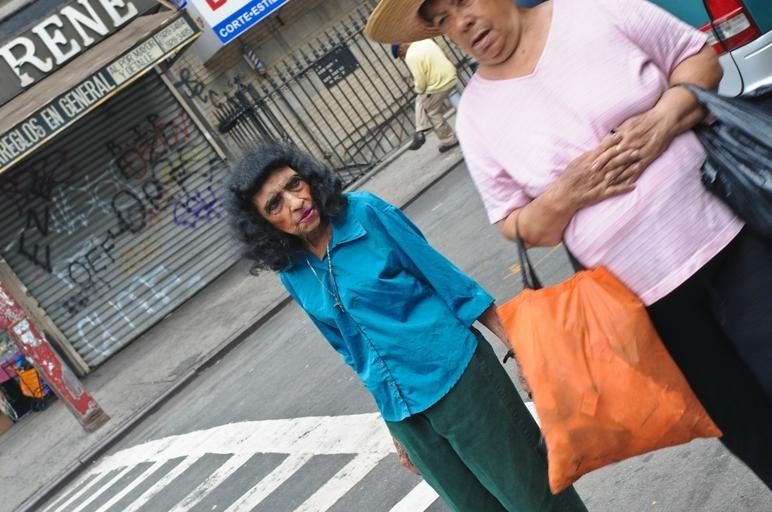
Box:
[[391, 43, 400, 60], [357, 0, 444, 43]]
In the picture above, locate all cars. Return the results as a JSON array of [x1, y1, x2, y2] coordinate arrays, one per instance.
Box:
[[648, 1, 772, 98]]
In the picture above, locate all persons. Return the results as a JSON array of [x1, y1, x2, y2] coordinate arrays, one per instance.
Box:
[[224, 141, 589, 511], [366, 0, 772, 511]]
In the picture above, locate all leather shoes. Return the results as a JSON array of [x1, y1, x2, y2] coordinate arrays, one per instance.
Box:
[[438, 140, 458, 153], [408, 132, 425, 150]]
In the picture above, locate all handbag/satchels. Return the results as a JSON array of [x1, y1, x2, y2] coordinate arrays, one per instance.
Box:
[[495, 265, 724, 495]]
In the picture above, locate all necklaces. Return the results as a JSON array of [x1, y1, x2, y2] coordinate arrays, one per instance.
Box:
[[305, 242, 346, 314]]
